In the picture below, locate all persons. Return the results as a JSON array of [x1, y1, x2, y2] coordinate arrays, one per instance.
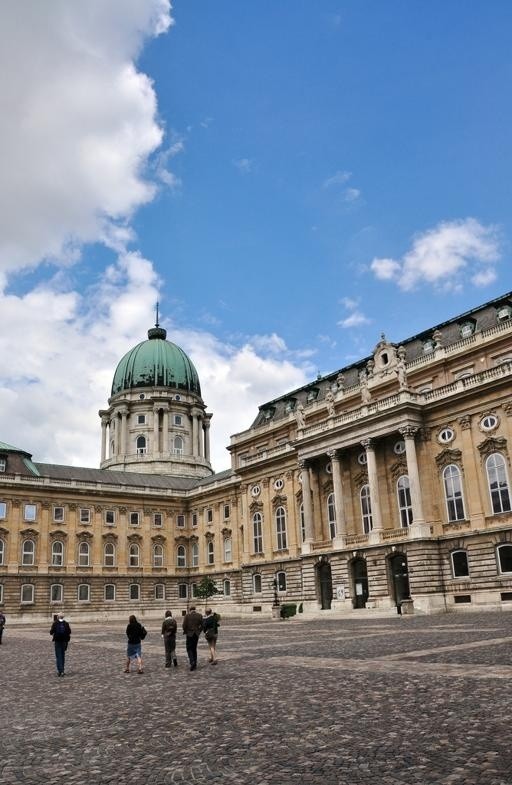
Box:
[[181, 606, 204, 671], [161, 611, 178, 668], [0, 611, 5, 645], [49, 612, 71, 677], [123, 615, 142, 674], [202, 608, 219, 665]]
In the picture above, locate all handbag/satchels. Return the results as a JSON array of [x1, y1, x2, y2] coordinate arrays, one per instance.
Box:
[[141, 625, 147, 639]]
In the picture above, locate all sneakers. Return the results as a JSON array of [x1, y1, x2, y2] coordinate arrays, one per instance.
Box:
[[165, 659, 218, 671], [57, 671, 65, 676]]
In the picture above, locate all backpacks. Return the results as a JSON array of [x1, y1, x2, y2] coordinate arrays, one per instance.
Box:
[[164, 620, 177, 636], [205, 616, 218, 641], [52, 620, 69, 642]]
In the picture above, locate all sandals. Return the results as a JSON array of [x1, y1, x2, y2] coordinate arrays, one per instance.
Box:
[[124, 668, 143, 673]]
